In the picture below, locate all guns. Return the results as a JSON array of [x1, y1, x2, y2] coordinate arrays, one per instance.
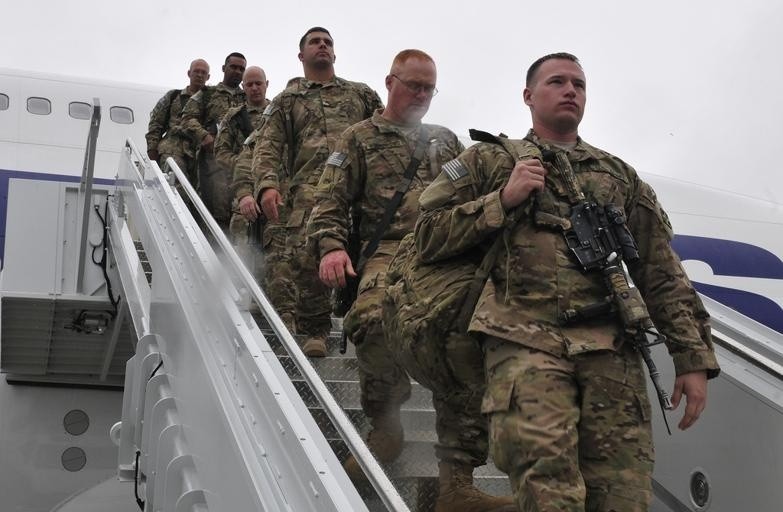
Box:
[[534, 148, 675, 412]]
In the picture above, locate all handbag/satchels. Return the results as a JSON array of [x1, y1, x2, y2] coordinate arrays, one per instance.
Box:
[[333, 232, 363, 316], [382, 134, 523, 406]]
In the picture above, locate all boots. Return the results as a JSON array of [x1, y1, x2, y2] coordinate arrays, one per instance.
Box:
[[434, 461, 517, 512], [303, 331, 328, 357], [280, 312, 297, 334], [250, 303, 262, 315], [344, 407, 404, 479]]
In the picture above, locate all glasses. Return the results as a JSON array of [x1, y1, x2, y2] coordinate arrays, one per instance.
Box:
[[392, 75, 437, 97]]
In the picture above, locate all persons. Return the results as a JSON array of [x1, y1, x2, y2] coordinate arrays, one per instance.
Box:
[[250, 27, 384, 359], [213, 64, 275, 276], [143, 57, 212, 200], [227, 76, 310, 261], [412, 47, 720, 508], [180, 52, 247, 210], [302, 47, 511, 510]]
